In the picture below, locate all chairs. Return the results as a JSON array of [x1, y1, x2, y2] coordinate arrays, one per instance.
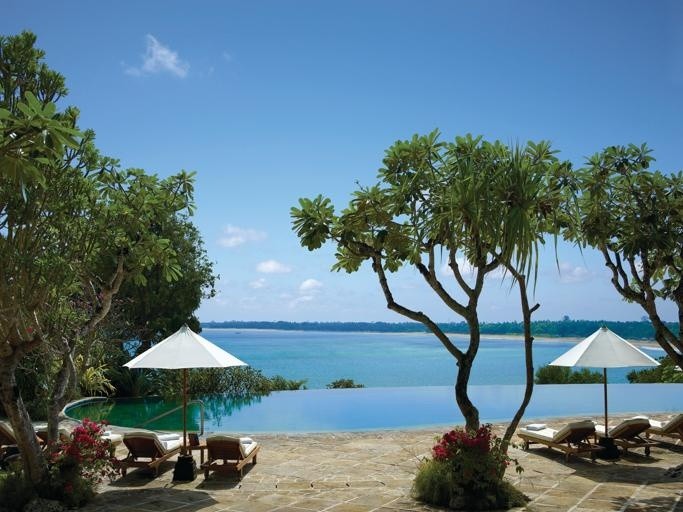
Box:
[[1, 420, 18, 446], [110, 430, 182, 479], [34, 425, 71, 441], [590, 415, 660, 458], [516, 419, 606, 464], [624, 412, 683, 444], [198, 434, 262, 478]]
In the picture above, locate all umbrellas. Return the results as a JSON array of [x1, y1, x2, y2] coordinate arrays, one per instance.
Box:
[[122, 323, 248, 455], [548, 325, 662, 437]]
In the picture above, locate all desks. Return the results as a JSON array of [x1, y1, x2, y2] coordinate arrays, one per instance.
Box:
[[180, 438, 208, 470]]
